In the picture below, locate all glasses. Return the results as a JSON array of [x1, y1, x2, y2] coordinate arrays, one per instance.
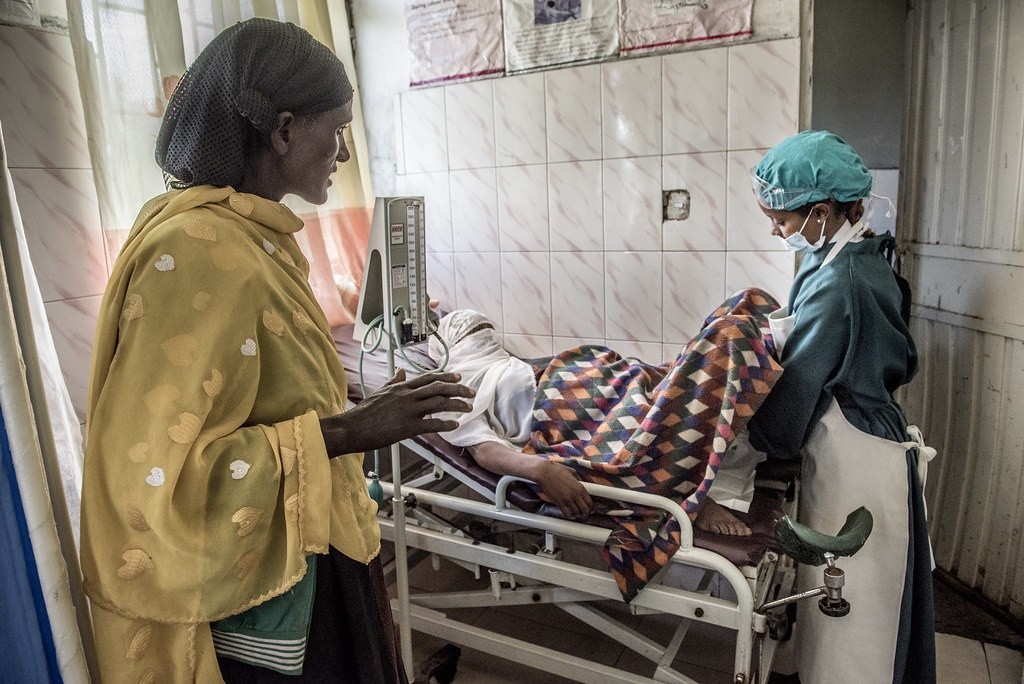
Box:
[[749, 159, 837, 209]]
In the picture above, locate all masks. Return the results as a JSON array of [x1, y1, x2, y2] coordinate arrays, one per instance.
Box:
[[776, 206, 827, 254]]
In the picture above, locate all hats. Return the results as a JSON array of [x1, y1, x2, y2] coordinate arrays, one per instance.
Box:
[[752, 130, 872, 210]]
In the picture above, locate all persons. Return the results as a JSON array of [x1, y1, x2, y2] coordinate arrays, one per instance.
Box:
[[82, 18, 477, 684], [429, 287, 797, 537], [746, 129, 937, 684]]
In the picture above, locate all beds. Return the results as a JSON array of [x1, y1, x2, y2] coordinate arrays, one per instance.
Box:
[[329, 322, 874, 684]]
